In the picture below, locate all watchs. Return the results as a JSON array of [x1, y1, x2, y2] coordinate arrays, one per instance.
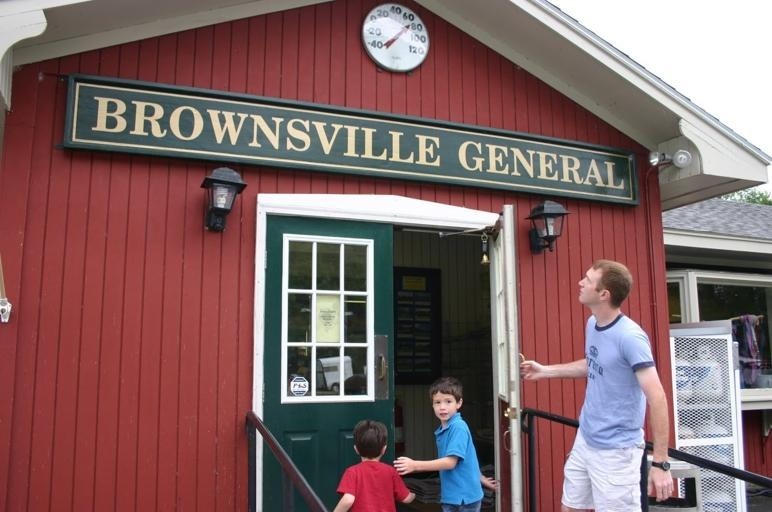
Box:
[[651, 460, 672, 471]]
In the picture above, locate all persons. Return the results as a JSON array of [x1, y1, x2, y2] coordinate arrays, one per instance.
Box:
[[333, 418, 416, 512], [392, 377, 501, 512], [521, 259, 675, 512]]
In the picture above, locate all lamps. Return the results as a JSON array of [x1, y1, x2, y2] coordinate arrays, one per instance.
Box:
[[523, 199, 573, 254], [199, 166, 248, 232], [647, 149, 691, 168]]
